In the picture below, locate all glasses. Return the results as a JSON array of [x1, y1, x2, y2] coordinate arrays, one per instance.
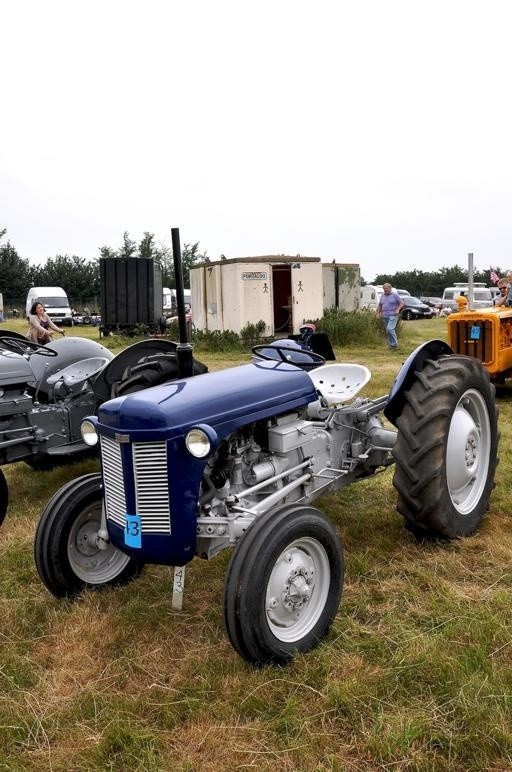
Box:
[[499, 286, 507, 290]]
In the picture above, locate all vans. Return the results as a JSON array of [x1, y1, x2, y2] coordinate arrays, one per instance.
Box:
[[25, 286, 74, 327], [441, 286, 494, 313]]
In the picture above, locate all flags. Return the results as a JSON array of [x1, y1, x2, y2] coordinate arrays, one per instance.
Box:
[[490, 268, 500, 285]]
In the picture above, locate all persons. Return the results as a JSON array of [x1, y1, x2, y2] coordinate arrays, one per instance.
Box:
[[25, 302, 65, 346], [493, 271, 512, 307], [376, 283, 405, 349]]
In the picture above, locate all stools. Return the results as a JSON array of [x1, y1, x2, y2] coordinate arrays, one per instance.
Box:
[[44, 355, 109, 400], [308, 362, 373, 426]]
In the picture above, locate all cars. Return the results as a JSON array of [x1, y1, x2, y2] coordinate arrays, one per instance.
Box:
[[400, 296, 434, 320]]
